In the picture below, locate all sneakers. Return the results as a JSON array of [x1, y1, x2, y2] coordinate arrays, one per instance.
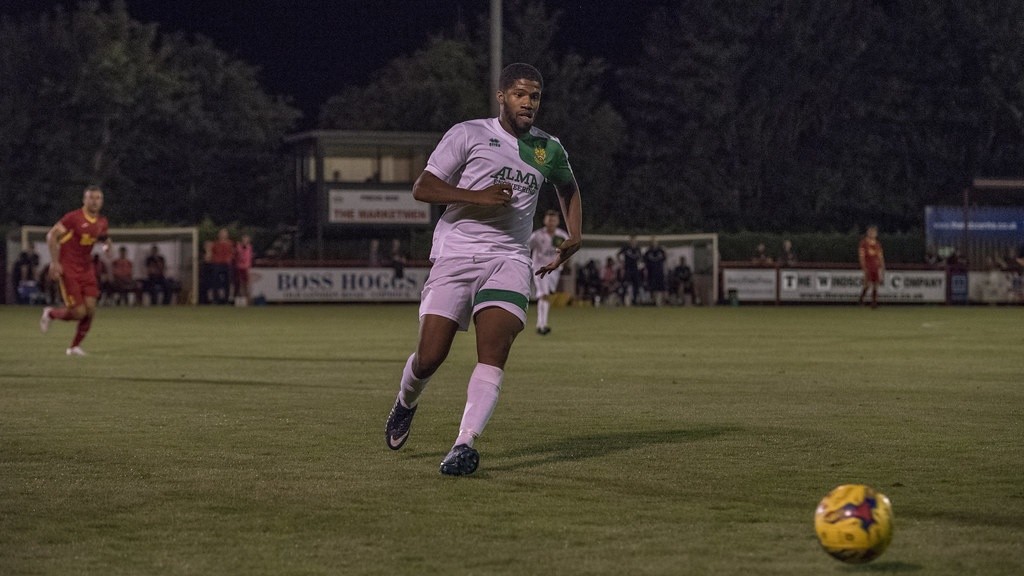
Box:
[[535, 325, 553, 336], [65, 344, 87, 357], [40, 306, 51, 335], [384, 392, 417, 451], [438, 444, 479, 477]]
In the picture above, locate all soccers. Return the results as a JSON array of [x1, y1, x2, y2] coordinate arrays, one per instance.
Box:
[[813, 483, 895, 563]]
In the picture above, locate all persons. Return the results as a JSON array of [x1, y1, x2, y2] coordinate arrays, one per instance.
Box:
[[385, 64, 583, 475], [364, 210, 800, 332], [10, 184, 253, 355], [857, 225, 886, 308]]
